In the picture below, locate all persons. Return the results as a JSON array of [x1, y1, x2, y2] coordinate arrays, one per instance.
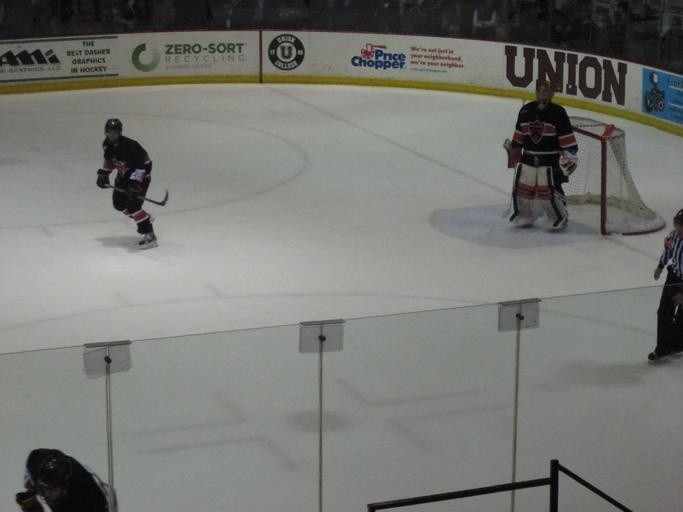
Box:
[[648, 208, 683, 361], [97, 118, 156, 246], [15, 448, 110, 512], [503, 77, 579, 230]]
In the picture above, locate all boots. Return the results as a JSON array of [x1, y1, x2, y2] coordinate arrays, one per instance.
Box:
[[647, 345, 670, 361], [138, 231, 157, 245]]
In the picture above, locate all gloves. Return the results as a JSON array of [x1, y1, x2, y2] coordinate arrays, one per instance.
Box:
[[128, 182, 142, 193], [503, 140, 522, 167], [556, 152, 578, 176], [97, 175, 110, 188], [16, 489, 40, 508]]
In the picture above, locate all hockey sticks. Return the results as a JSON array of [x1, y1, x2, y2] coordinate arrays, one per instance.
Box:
[[104, 182, 169, 206], [502, 158, 516, 218]]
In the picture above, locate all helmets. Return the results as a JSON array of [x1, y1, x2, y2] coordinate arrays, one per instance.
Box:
[[39, 454, 69, 489], [106, 119, 122, 129], [673, 208, 683, 224], [535, 81, 554, 102]]
